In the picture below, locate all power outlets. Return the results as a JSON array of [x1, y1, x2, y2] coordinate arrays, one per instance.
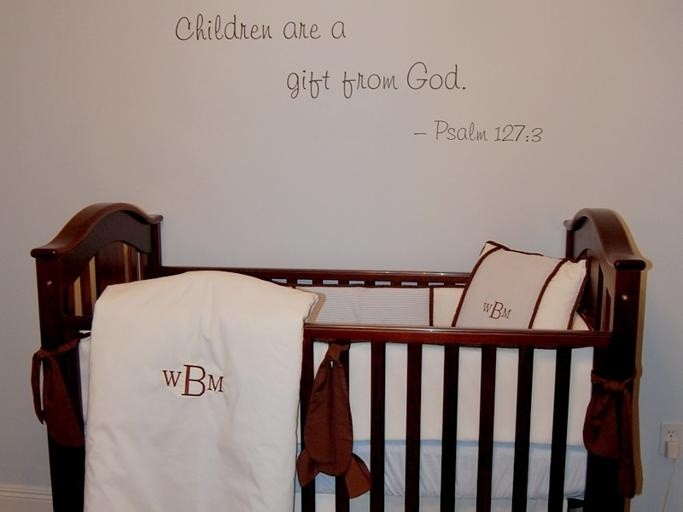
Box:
[[658, 421, 683, 457]]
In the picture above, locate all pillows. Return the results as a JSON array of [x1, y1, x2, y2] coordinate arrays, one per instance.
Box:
[[451, 241, 589, 331]]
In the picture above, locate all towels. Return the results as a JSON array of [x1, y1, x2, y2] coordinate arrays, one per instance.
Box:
[[83, 271, 319, 512]]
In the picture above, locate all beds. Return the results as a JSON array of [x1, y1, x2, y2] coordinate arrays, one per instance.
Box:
[[30, 202, 654, 511]]
